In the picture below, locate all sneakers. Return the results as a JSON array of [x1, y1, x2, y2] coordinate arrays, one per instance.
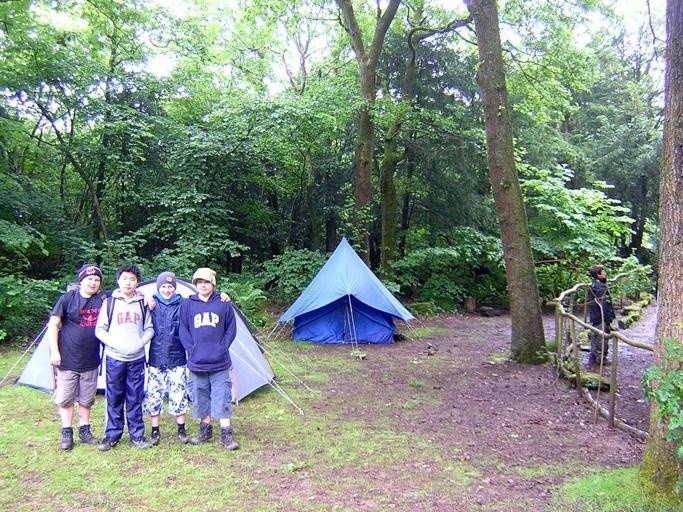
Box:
[[600, 357, 611, 365], [178, 428, 190, 444], [151, 431, 161, 445], [130, 436, 153, 449], [99, 434, 119, 451]]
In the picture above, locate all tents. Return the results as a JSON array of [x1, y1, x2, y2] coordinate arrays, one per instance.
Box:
[[277, 238, 415, 345], [17, 278, 275, 404]]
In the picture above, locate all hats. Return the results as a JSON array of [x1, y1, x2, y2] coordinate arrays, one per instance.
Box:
[[192, 267, 217, 286], [78, 263, 103, 282], [156, 271, 176, 291]]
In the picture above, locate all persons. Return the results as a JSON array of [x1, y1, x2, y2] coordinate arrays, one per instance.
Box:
[[178, 267, 239, 450], [143, 271, 230, 446], [587, 266, 615, 364], [47, 264, 157, 449], [95, 266, 155, 451]]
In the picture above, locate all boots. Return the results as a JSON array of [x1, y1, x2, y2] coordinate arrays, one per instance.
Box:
[[191, 423, 214, 445], [78, 424, 99, 444], [57, 427, 74, 450], [220, 425, 239, 450]]
